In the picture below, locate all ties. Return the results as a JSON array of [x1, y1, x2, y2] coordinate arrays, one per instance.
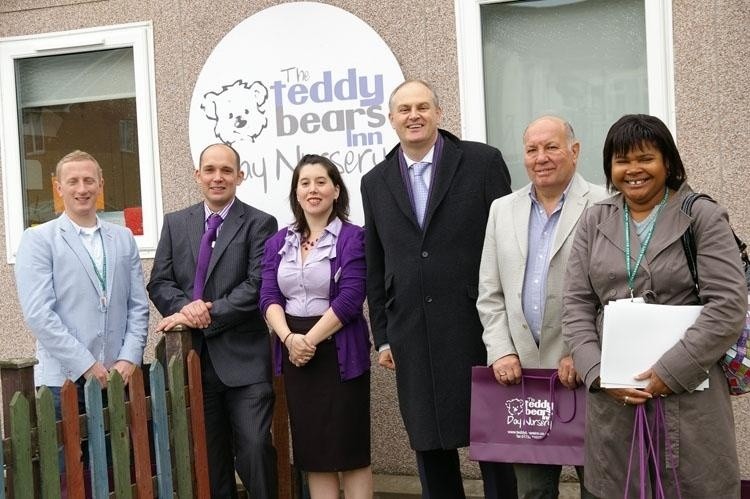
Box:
[[410, 162, 432, 229], [194, 213, 223, 303]]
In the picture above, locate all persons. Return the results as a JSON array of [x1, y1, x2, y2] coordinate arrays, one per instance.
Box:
[[476, 117, 620, 499], [562, 115, 748, 499], [360, 81, 517, 499], [261, 155, 374, 499], [146, 144, 278, 498], [14, 150, 149, 475]]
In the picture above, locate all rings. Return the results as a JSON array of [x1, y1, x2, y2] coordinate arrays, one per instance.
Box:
[[499, 372, 506, 376], [661, 394, 667, 397], [624, 403, 626, 406], [625, 396, 628, 403]]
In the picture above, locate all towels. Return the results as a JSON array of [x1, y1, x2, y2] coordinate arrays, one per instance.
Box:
[[471, 366, 588, 466]]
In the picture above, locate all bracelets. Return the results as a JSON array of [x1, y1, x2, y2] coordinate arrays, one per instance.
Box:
[[283, 332, 291, 345]]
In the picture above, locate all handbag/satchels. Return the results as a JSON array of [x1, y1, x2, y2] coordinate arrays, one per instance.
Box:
[[680, 192, 750, 395]]
